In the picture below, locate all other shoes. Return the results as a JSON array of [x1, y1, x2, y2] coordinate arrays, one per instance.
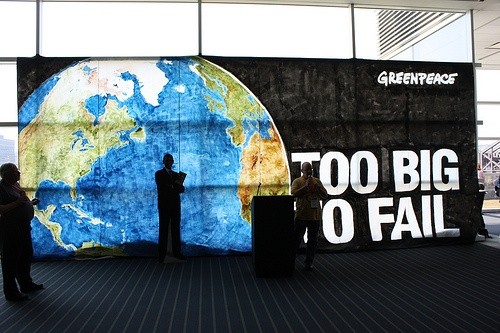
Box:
[[305, 264, 313, 269], [7, 284, 43, 299]]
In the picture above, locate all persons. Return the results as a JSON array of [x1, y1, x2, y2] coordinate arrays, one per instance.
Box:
[[291, 162, 328, 271], [155, 154, 185, 259], [0, 163, 43, 301]]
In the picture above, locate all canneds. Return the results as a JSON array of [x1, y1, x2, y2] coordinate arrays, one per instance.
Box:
[[20, 189, 25, 199]]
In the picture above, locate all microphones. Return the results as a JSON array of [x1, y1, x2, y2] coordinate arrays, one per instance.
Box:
[[308, 175, 312, 189], [256, 183, 261, 196]]
